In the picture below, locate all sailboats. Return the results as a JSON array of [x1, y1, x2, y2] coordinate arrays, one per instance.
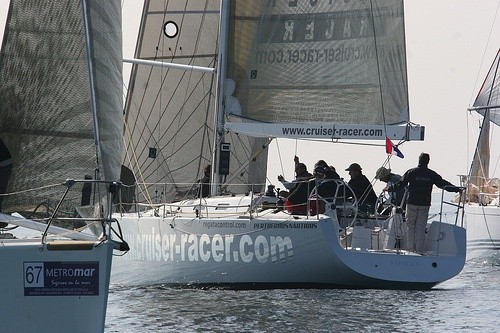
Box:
[[77, 0, 467, 290], [427, 46, 500, 263], [0, 1, 130, 333]]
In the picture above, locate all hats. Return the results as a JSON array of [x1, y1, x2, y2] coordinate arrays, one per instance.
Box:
[[374, 166, 392, 179], [344, 163, 363, 172]]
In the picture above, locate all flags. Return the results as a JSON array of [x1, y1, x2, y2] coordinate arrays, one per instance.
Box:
[[385, 136, 404, 158]]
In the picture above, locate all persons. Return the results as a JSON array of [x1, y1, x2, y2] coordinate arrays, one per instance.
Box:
[[338, 163, 377, 215], [195, 163, 211, 199], [382, 154, 462, 252], [375, 167, 409, 248], [274, 155, 344, 216]]
